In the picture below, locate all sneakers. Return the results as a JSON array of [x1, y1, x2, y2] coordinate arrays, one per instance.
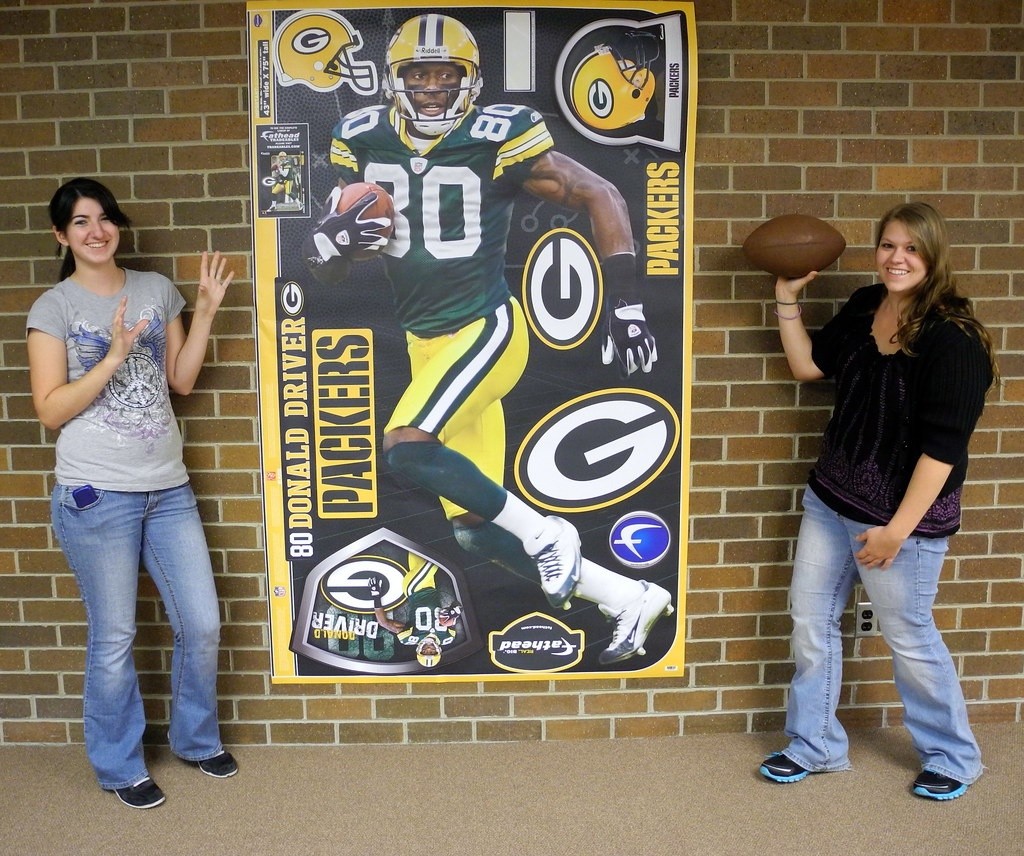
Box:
[[167, 729, 239, 778], [597, 579, 674, 666], [297, 203, 303, 210], [266, 206, 277, 213], [522, 515, 581, 611], [760, 752, 811, 783], [112, 775, 165, 809], [913, 771, 967, 801]]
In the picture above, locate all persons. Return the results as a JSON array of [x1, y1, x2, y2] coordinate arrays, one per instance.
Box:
[[304, 13, 676, 666], [263, 152, 305, 216], [24, 176, 240, 808], [759, 202, 983, 802]]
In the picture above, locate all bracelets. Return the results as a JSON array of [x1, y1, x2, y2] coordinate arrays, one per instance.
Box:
[[773, 299, 802, 321]]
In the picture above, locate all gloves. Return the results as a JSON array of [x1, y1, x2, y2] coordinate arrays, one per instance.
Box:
[[301, 187, 391, 271], [439, 603, 461, 624], [368, 577, 384, 604], [601, 291, 660, 379]]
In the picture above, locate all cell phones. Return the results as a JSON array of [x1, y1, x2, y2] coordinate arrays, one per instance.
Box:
[[72, 485, 97, 508]]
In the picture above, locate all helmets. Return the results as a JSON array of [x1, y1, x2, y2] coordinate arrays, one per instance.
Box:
[[278, 152, 288, 160], [416, 639, 442, 667], [383, 14, 478, 137]]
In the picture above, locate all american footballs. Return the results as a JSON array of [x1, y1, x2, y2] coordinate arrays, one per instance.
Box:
[[742, 214, 847, 279], [336, 182, 394, 262]]
